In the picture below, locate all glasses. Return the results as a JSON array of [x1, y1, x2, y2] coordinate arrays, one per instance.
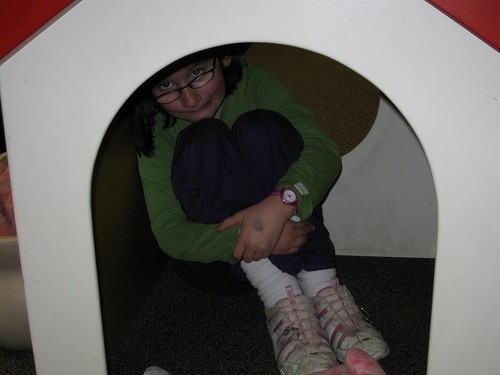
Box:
[[148, 58, 216, 104]]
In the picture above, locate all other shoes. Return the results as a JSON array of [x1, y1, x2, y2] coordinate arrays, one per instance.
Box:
[[309, 278, 391, 363], [264, 286, 340, 375]]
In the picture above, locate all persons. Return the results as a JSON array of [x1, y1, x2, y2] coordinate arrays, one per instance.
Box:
[[127, 52, 390, 375]]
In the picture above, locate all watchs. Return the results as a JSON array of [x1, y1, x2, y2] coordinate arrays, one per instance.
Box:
[[272, 187, 301, 223]]
